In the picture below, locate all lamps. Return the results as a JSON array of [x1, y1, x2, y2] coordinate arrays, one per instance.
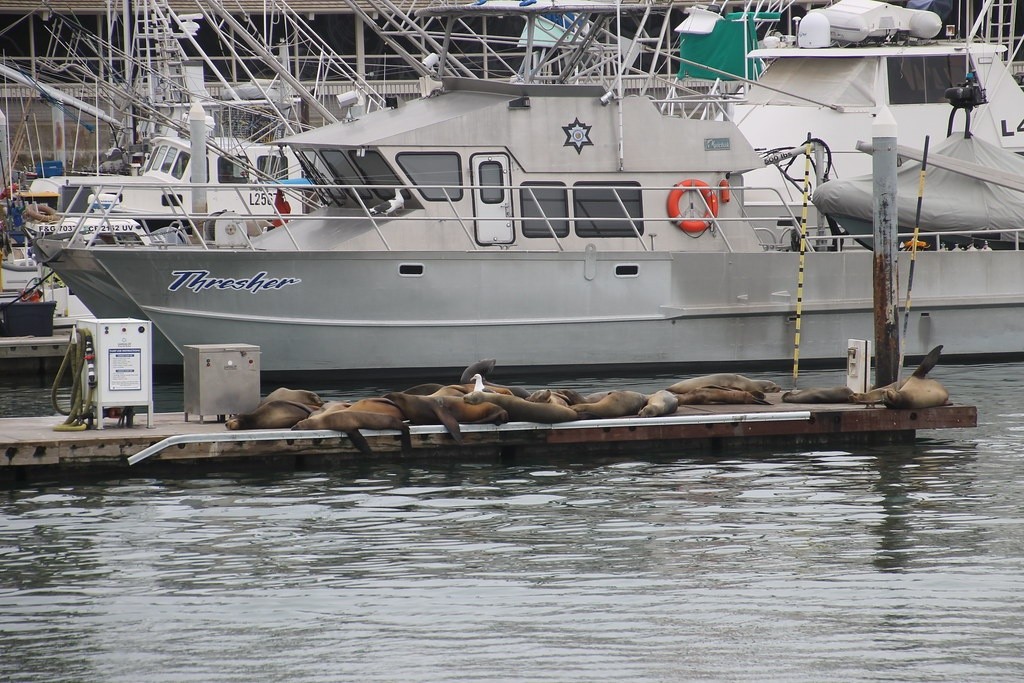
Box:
[[421, 54, 439, 75], [337, 90, 358, 123]]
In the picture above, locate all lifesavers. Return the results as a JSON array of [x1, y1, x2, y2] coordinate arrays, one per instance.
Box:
[[667, 179, 718, 232]]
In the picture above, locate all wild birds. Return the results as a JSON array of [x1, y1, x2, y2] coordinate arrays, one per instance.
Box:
[[469, 373, 494, 393], [368, 188, 406, 216]]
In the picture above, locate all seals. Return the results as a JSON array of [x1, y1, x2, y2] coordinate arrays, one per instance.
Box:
[[223, 345, 949, 460]]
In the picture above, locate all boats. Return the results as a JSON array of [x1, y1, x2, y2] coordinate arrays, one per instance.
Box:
[[14, 102, 318, 248], [26, 0, 1024, 391], [713, 0, 1024, 251]]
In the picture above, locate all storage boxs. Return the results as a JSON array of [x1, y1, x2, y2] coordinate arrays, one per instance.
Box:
[[0, 301, 57, 337]]
